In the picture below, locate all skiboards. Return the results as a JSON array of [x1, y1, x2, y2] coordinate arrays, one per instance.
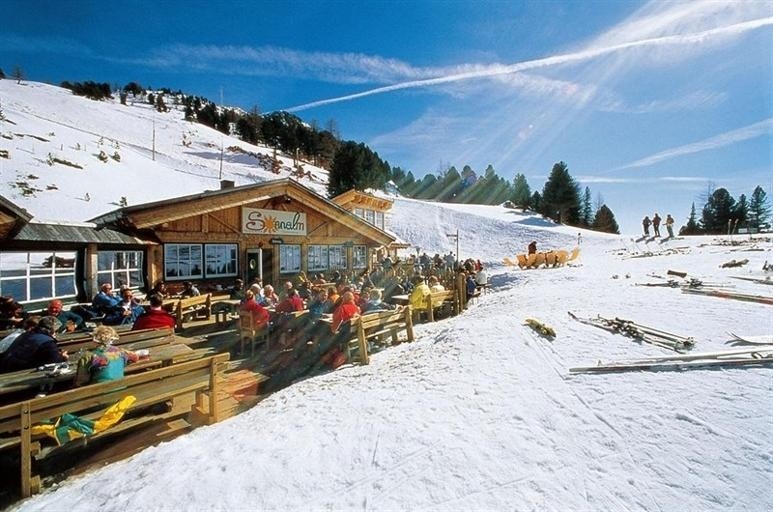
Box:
[[569, 312, 773, 375], [637, 282, 736, 289]]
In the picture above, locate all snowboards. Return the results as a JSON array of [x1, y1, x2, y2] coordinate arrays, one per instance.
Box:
[[526, 318, 555, 337]]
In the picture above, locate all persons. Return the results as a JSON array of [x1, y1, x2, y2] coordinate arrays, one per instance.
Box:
[[92, 280, 201, 331], [231, 252, 488, 365], [662, 215, 674, 237], [1, 295, 84, 371], [74, 326, 138, 411], [529, 241, 537, 254], [652, 214, 662, 237], [642, 216, 651, 234]]
